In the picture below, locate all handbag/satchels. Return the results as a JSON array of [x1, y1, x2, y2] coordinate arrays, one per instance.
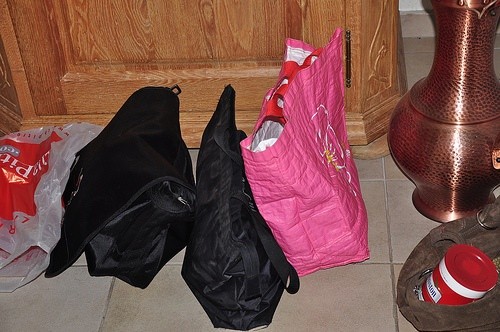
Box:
[[397, 194, 500, 332], [0, 121, 104, 295], [180, 85, 300, 331], [239, 27, 371, 277], [44, 84, 196, 290]]
